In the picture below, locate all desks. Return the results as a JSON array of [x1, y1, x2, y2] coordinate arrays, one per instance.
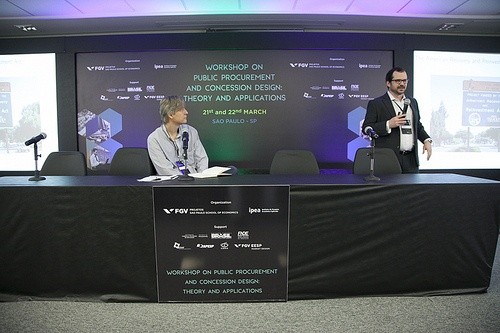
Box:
[[0, 173, 500, 302]]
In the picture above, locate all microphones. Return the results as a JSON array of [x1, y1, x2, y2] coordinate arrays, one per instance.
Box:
[[364, 127, 379, 139], [182, 132, 189, 150], [25, 133, 47, 146], [401, 99, 411, 118]]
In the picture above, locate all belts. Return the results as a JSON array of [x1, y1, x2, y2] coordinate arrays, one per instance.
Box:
[[400, 150, 413, 155]]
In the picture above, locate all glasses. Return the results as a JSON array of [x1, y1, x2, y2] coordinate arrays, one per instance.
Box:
[[391, 79, 408, 84]]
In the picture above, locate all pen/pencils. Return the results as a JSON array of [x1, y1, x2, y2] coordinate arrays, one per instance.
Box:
[[397, 112, 400, 128]]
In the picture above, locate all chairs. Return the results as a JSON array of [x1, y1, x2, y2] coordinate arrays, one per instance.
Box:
[[353, 147, 403, 174], [108, 147, 152, 175], [269, 149, 320, 175], [38, 151, 88, 177]]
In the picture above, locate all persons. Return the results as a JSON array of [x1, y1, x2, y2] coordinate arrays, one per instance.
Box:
[[90, 147, 102, 169], [147, 96, 208, 175], [362, 67, 432, 174]]
